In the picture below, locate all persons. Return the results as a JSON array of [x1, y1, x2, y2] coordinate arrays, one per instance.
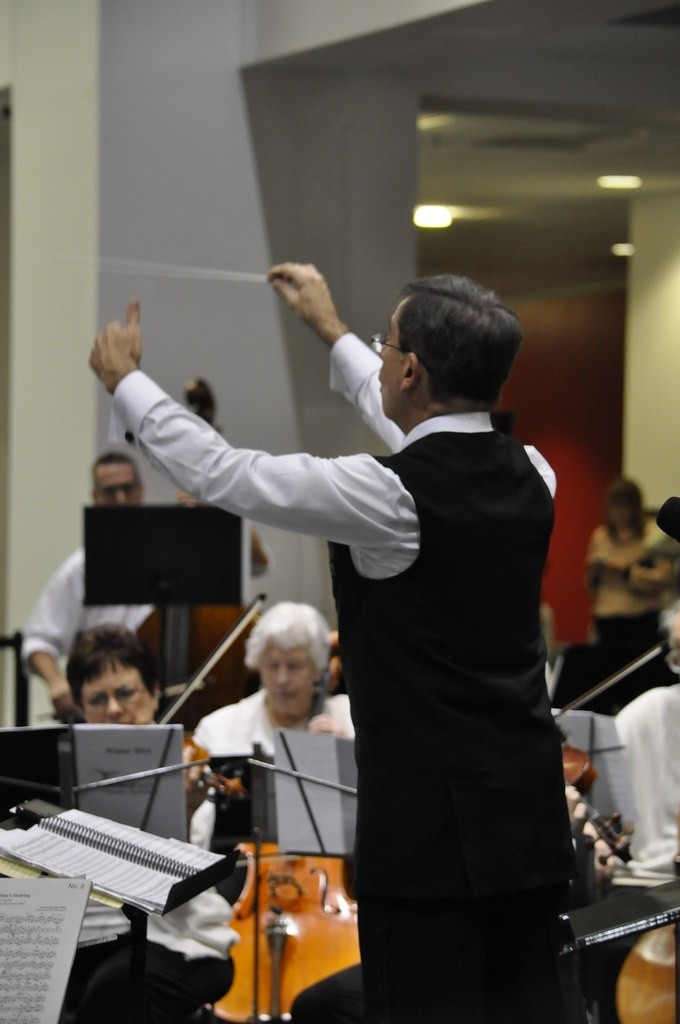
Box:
[[185, 599, 354, 911], [548, 478, 680, 1024], [57, 620, 242, 1023], [22, 452, 155, 722], [86, 260, 577, 1024]]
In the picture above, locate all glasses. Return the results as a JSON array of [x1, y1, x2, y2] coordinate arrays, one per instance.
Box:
[[96, 484, 136, 494], [371, 333, 409, 354]]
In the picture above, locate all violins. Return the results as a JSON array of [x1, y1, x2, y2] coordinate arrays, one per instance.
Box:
[[176, 734, 246, 798], [563, 747, 636, 856]]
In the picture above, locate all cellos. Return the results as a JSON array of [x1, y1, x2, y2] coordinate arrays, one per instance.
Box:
[[613, 919, 676, 1024], [129, 380, 271, 742], [205, 827, 381, 1024]]
